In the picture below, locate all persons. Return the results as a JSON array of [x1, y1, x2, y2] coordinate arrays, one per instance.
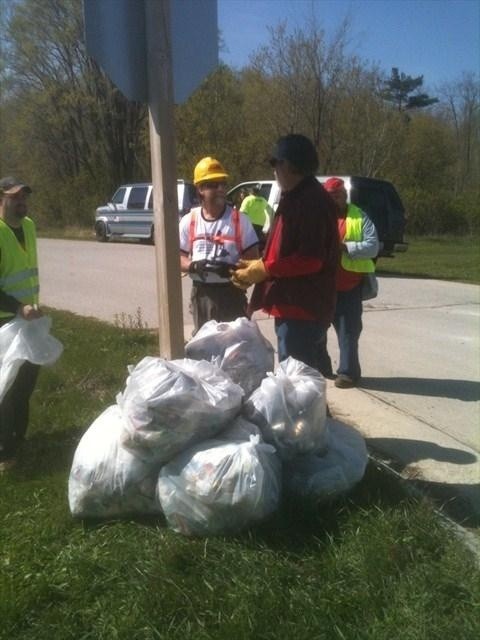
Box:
[[1, 176, 66, 467], [229, 133, 342, 415], [178, 155, 262, 344], [238, 186, 269, 257], [324, 177, 380, 388], [239, 188, 248, 200]]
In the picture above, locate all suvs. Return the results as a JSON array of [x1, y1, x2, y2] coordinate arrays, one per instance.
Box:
[[225, 175, 409, 263], [94, 179, 198, 245]]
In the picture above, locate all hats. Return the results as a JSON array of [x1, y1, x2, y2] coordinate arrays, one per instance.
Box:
[[269, 134, 318, 178], [0, 176, 32, 194]]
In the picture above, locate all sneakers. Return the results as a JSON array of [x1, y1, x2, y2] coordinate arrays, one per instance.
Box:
[[335, 374, 353, 388]]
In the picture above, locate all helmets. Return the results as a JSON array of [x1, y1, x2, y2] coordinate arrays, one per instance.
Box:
[[322, 177, 344, 196], [193, 157, 228, 185]]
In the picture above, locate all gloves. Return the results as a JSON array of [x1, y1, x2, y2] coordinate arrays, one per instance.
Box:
[[229, 257, 268, 289], [189, 259, 237, 283]]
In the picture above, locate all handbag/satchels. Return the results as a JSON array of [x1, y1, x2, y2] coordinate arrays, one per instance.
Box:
[[361, 272, 378, 300]]
